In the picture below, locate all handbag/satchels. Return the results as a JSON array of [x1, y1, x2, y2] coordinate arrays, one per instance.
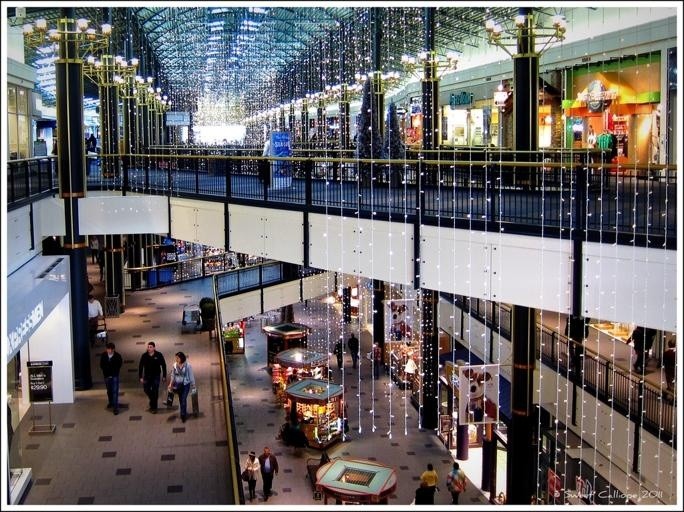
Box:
[[172, 381, 184, 394], [241, 469, 250, 481], [167, 389, 174, 407]]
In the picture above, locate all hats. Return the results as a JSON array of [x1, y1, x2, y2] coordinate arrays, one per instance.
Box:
[[249, 451, 256, 458]]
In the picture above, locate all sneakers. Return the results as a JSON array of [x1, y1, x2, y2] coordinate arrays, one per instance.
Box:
[[107, 403, 119, 415], [179, 414, 186, 423], [144, 406, 156, 414]]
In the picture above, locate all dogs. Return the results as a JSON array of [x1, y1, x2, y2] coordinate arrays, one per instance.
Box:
[[462, 367, 497, 423], [387, 302, 412, 341]]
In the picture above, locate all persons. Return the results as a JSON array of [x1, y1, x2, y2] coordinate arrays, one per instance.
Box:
[[85, 138, 89, 154], [258, 447, 279, 501], [244, 451, 261, 503], [90, 236, 99, 265], [100, 343, 122, 415], [261, 139, 271, 157], [347, 333, 359, 369], [587, 124, 597, 146], [88, 295, 104, 349], [320, 447, 330, 466], [420, 463, 438, 487], [138, 342, 167, 414], [446, 462, 465, 504], [162, 352, 196, 422], [415, 482, 435, 505], [334, 338, 346, 368], [89, 134, 97, 152]]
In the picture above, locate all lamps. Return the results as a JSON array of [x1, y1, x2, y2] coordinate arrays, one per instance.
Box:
[[22, 8, 154, 110], [355, 7, 567, 105]]
